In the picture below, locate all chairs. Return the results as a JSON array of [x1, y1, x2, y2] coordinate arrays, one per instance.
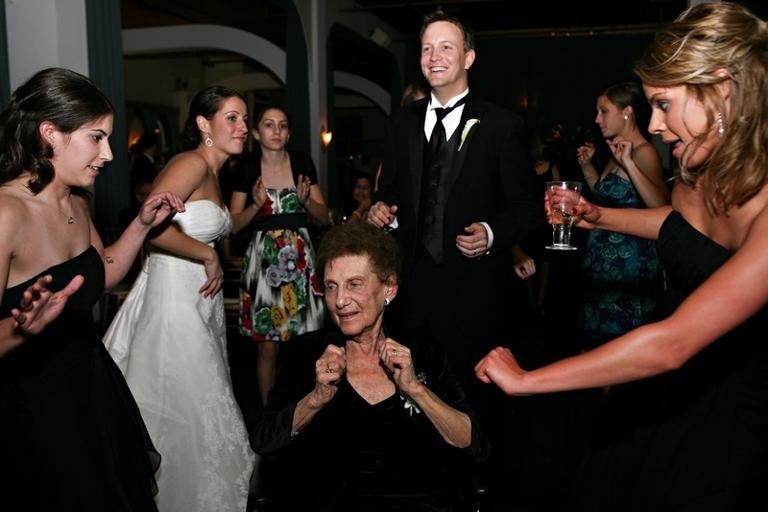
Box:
[[99, 234, 249, 335]]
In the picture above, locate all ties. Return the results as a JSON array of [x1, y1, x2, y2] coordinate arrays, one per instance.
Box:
[[421, 95, 466, 263]]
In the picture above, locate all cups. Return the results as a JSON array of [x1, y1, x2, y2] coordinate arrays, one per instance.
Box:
[[544, 180, 577, 250]]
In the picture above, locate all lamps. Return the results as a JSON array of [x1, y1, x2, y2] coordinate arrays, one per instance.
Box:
[[321, 125, 335, 148]]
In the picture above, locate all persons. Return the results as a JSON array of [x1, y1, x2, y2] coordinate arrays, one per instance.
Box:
[[1, 2, 767, 512]]
